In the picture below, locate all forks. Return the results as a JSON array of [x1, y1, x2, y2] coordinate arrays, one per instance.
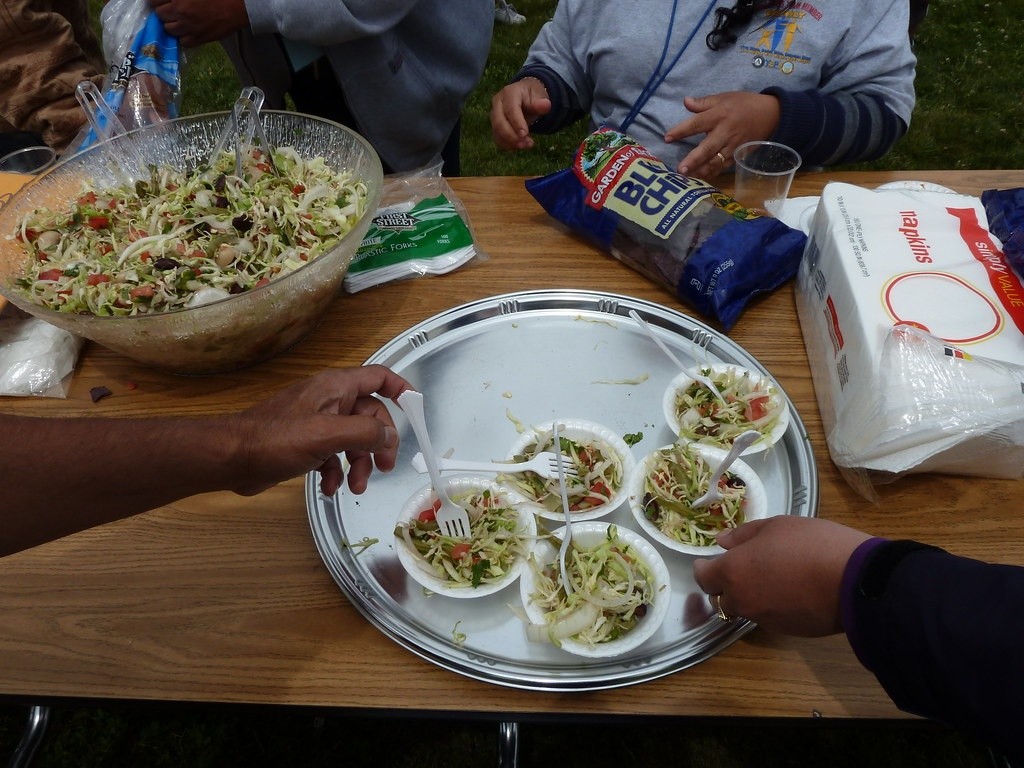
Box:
[[397, 388, 471, 541], [553, 422, 576, 596], [408, 449, 578, 479], [690, 429, 763, 512], [629, 309, 724, 402]]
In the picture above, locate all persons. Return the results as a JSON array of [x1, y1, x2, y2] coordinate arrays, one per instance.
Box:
[[144, 0, 930, 183], [0, 362, 417, 559], [693, 516, 1024, 740]]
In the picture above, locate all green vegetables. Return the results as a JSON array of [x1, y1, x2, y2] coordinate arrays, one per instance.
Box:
[[12, 146, 366, 319], [410, 368, 783, 648]]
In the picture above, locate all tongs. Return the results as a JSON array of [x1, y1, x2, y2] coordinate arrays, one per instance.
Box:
[[74, 80, 148, 192], [210, 83, 264, 174], [229, 96, 281, 182]]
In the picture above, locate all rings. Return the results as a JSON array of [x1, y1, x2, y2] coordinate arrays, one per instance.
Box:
[[715, 595, 730, 621], [717, 153, 726, 164]]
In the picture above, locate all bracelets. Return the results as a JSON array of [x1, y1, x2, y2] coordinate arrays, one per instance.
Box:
[[519, 77, 548, 125]]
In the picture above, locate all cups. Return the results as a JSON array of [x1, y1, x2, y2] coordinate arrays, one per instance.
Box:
[[0, 145, 58, 190], [732, 141, 802, 221]]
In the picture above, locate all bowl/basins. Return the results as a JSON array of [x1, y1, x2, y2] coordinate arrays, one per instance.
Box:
[[504, 419, 636, 523], [520, 520, 672, 659], [2, 110, 384, 374], [629, 441, 768, 556], [662, 364, 791, 457], [394, 474, 537, 598]]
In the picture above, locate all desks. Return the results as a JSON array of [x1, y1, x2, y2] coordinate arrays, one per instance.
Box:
[[0, 169, 1024, 725]]
